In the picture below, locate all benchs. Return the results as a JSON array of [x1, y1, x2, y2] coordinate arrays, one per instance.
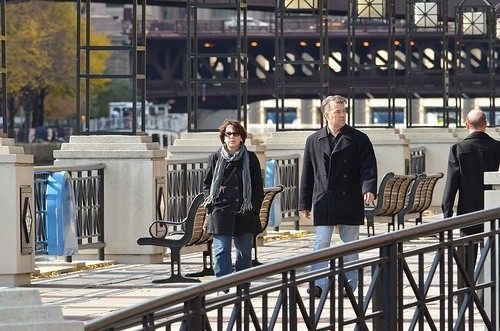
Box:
[[137, 184, 285, 283], [363, 172, 445, 237]]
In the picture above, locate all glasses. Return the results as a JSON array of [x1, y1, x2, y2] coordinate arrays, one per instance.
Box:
[[225, 132, 239, 137]]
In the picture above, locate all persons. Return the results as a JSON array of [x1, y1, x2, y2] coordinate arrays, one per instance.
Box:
[[298, 94, 378, 297], [441, 110, 500, 309], [202, 119, 265, 293]]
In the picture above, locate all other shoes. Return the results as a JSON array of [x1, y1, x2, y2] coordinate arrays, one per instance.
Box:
[[223, 289, 229, 294], [307, 286, 322, 298]]
